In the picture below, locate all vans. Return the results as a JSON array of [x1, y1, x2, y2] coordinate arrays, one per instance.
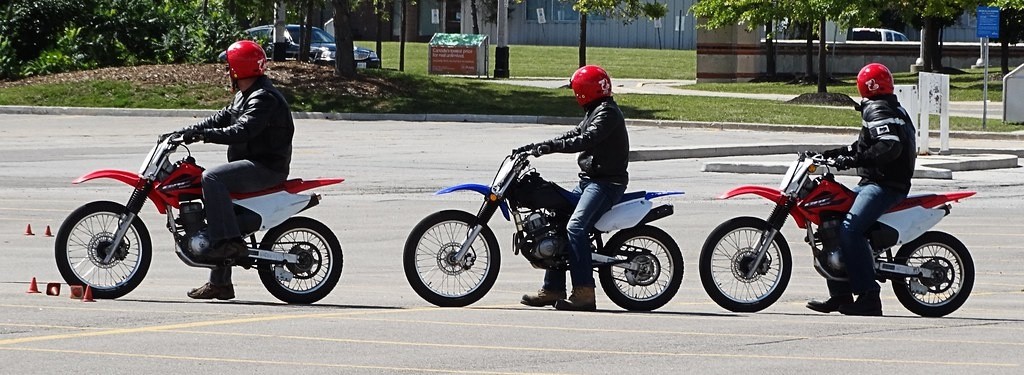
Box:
[[852, 28, 909, 41]]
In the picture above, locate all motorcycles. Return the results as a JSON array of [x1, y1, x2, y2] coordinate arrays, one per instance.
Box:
[[403, 147, 684, 311], [699, 154, 977, 318], [55, 133, 344, 306]]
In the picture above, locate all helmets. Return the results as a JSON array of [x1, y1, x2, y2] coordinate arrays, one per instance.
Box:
[[558, 64, 613, 107], [216, 40, 270, 94], [856, 62, 895, 100]]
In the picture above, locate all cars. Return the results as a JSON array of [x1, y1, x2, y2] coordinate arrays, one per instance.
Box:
[[218, 24, 380, 69]]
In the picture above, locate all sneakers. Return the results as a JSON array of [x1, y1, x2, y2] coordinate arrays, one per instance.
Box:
[[187, 281, 236, 301], [806, 298, 855, 316], [203, 238, 250, 265], [838, 293, 883, 317], [555, 287, 598, 311], [522, 287, 570, 306]]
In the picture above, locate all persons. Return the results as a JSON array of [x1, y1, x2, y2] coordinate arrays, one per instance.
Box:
[[168, 40, 294, 300], [517, 65, 629, 312], [806, 63, 916, 317]]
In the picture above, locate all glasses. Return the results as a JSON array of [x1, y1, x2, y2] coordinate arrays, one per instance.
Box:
[[226, 65, 231, 71]]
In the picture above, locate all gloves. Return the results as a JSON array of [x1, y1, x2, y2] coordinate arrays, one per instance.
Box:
[[184, 128, 204, 146], [155, 131, 178, 146], [799, 149, 817, 163], [531, 141, 557, 158], [834, 155, 855, 172], [509, 143, 535, 160]]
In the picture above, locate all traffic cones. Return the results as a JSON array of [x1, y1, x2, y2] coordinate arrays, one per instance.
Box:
[[27, 277, 41, 293], [25, 224, 32, 235], [70, 285, 84, 299], [81, 284, 96, 302], [45, 225, 53, 237], [47, 283, 61, 295]]
